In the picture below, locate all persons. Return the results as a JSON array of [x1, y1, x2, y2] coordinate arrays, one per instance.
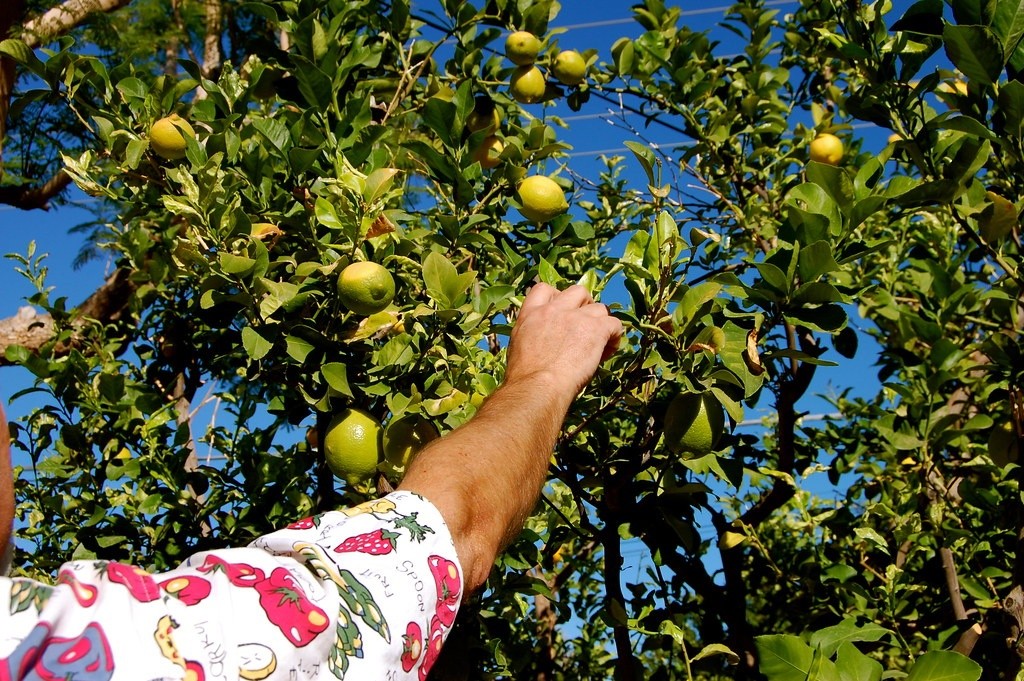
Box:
[[0, 283, 620, 681]]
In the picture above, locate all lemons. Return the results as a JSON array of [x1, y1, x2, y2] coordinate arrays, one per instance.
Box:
[[148, 31, 845, 489]]
